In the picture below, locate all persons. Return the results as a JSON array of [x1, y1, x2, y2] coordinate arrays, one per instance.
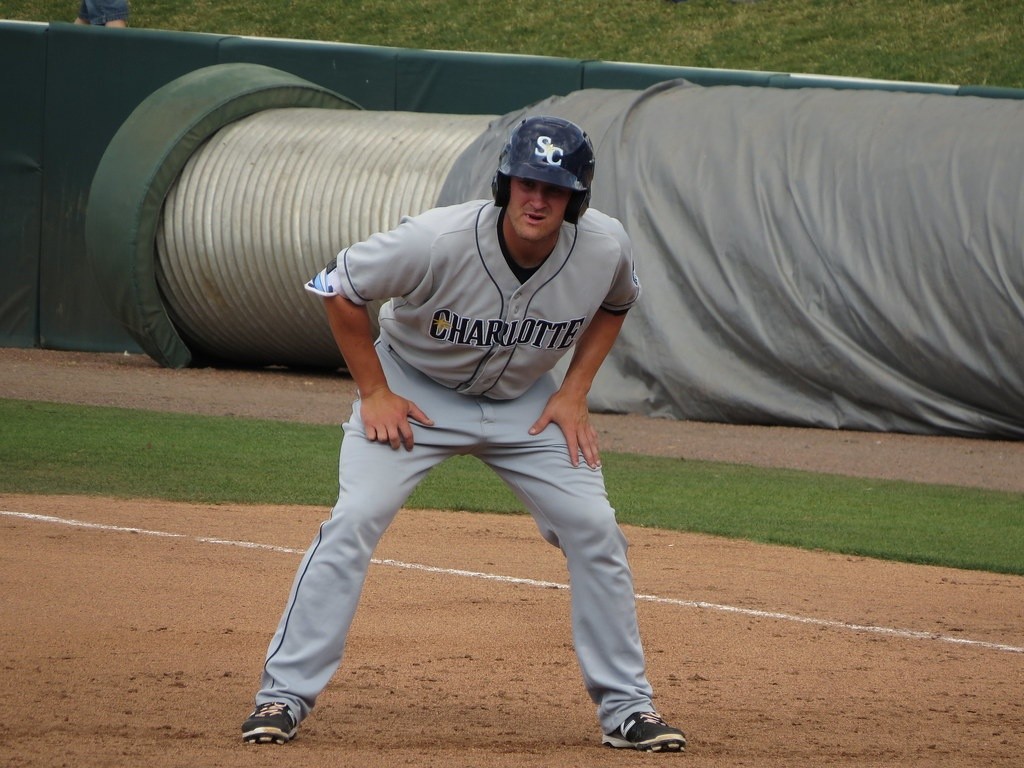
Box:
[[240, 116, 688, 754]]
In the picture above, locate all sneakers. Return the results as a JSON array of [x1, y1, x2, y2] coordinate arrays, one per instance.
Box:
[[602, 712, 687, 753], [241, 702, 299, 746]]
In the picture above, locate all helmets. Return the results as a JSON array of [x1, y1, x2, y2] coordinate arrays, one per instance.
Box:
[[491, 114, 595, 226]]
